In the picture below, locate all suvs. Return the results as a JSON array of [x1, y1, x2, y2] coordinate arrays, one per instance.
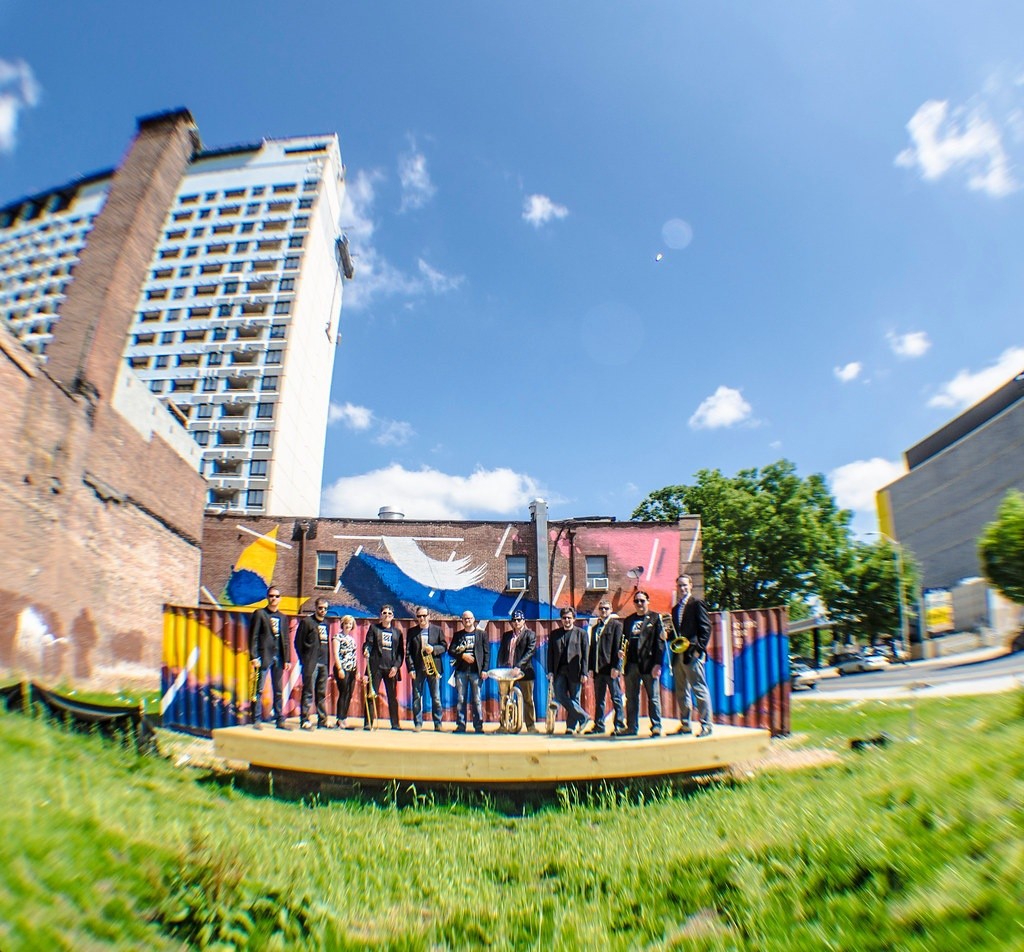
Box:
[[829, 652, 889, 675]]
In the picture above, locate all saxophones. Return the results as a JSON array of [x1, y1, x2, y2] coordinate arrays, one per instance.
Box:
[[544, 673, 559, 735]]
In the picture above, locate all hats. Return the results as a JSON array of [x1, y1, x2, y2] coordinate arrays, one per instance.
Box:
[[511, 610, 524, 619]]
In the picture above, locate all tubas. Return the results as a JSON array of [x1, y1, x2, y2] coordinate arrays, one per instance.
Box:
[[487, 667, 525, 735]]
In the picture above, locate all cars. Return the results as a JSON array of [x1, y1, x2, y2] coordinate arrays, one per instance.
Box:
[[789, 663, 821, 690]]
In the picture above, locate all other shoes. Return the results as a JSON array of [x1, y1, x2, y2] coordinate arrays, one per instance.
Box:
[[253, 719, 711, 738]]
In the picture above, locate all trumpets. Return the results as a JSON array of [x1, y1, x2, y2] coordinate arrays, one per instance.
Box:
[[248, 659, 259, 702], [620, 634, 629, 675], [420, 642, 442, 681]]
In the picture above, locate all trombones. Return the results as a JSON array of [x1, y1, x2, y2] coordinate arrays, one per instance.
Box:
[[361, 650, 378, 733], [658, 611, 690, 676]]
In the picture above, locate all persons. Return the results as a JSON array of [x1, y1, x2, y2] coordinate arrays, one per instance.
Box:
[[613, 591, 665, 738], [331, 615, 358, 730], [448, 610, 489, 733], [293, 598, 335, 730], [405, 607, 448, 732], [659, 574, 714, 738], [362, 604, 406, 731], [247, 586, 294, 731], [546, 606, 591, 734], [491, 610, 540, 734], [584, 600, 627, 737]]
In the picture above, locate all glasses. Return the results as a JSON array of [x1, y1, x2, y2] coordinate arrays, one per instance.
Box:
[[318, 607, 328, 611], [599, 607, 610, 611], [634, 599, 645, 603], [417, 615, 427, 618], [381, 612, 393, 615], [512, 618, 522, 622], [562, 617, 572, 620], [268, 594, 280, 598]]
